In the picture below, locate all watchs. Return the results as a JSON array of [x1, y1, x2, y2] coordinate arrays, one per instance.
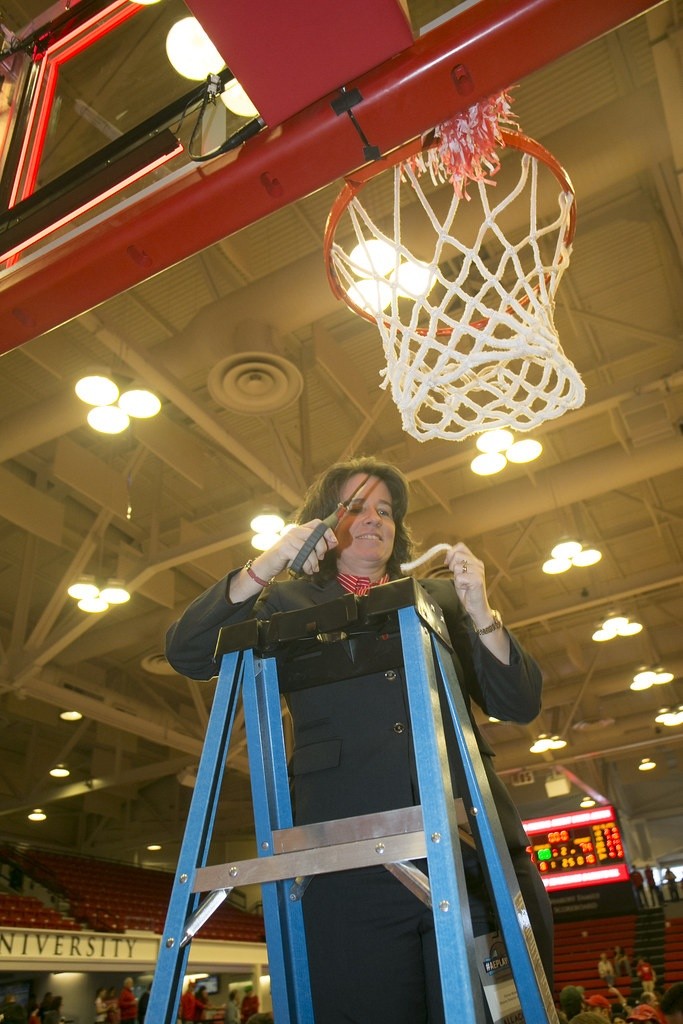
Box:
[[472, 609, 502, 636]]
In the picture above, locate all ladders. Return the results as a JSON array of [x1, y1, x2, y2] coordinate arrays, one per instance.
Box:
[[143, 577, 558, 1024]]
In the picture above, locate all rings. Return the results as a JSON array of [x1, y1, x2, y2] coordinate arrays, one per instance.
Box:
[[462, 560, 467, 573]]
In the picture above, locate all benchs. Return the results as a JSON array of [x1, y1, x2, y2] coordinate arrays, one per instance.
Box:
[[552, 914, 683, 1010]]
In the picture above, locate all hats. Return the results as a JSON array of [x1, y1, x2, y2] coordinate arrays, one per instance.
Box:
[[626, 1003, 661, 1023], [559, 986, 582, 1014], [584, 994, 611, 1009]]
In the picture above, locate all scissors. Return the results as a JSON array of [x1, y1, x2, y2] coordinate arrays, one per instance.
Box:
[[290, 473, 370, 579]]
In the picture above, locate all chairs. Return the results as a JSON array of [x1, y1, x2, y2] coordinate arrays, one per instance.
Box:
[[0, 846, 265, 941]]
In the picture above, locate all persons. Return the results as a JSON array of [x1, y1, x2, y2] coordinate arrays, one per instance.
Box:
[[179, 982, 214, 1024], [559, 983, 683, 1024], [631, 865, 648, 910], [612, 944, 630, 976], [636, 956, 657, 992], [665, 866, 679, 902], [598, 952, 614, 987], [94, 977, 152, 1024], [164, 456, 554, 1024], [0, 992, 65, 1024], [645, 864, 661, 907], [224, 990, 241, 1024], [240, 985, 260, 1024]]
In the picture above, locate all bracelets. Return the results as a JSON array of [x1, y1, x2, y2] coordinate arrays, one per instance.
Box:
[[245, 559, 274, 587]]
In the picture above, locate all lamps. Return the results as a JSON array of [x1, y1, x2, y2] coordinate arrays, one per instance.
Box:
[[529, 660, 683, 809], [469, 426, 544, 477], [27, 808, 48, 821], [541, 466, 604, 576], [74, 294, 162, 436], [591, 573, 643, 642], [49, 762, 72, 778], [165, 17, 260, 119], [345, 179, 436, 314], [68, 530, 131, 613], [250, 453, 303, 552], [58, 708, 83, 721]]
